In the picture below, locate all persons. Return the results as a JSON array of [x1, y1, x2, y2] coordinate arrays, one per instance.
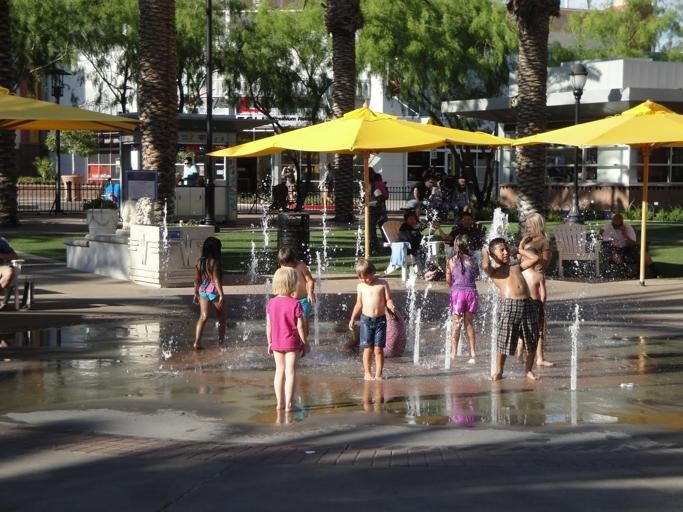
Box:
[[515, 246, 554, 367], [182, 156, 200, 185], [445, 234, 480, 360], [266, 267, 308, 412], [405, 174, 436, 219], [398, 210, 438, 282], [602, 213, 637, 273], [368, 162, 390, 248], [0, 237, 19, 290], [191, 236, 226, 350], [277, 246, 317, 337], [453, 176, 477, 213], [281, 161, 296, 200], [382, 306, 407, 357], [447, 211, 482, 251], [318, 161, 334, 216], [348, 257, 397, 381], [481, 238, 541, 381], [517, 212, 552, 282]]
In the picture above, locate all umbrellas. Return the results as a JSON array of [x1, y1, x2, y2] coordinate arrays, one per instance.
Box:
[[206, 100, 511, 265], [511, 99, 683, 287], [0, 84, 144, 133]]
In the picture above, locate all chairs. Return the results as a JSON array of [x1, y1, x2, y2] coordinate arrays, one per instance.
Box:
[[553, 224, 604, 278], [451, 222, 492, 279], [381, 220, 448, 279], [0, 258, 38, 311]]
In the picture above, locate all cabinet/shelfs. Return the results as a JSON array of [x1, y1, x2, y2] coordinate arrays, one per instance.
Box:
[[173, 186, 195, 217], [196, 186, 227, 218]]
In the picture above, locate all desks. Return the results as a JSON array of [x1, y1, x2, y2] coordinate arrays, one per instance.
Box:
[[419, 240, 449, 273]]
